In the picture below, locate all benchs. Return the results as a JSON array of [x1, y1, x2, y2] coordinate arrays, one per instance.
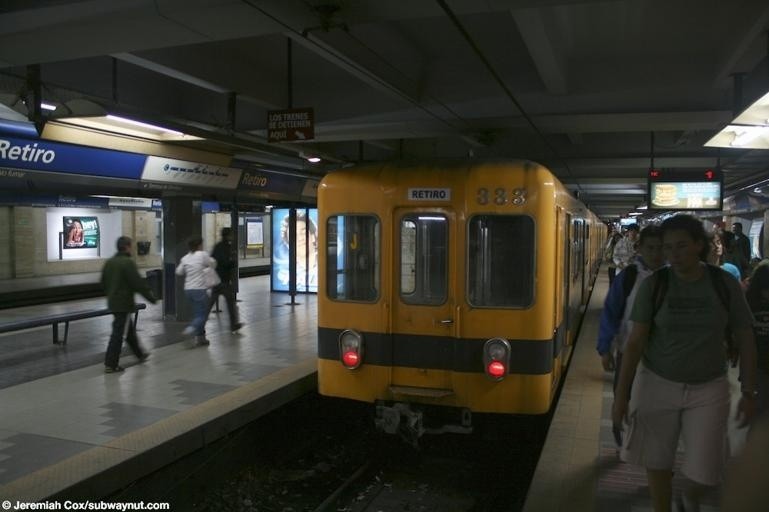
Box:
[[0, 303, 146, 345]]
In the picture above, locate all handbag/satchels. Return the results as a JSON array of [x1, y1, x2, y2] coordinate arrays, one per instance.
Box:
[[203, 267, 221, 288]]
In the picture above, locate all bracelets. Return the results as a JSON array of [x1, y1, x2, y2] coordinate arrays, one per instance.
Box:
[[742, 388, 762, 398]]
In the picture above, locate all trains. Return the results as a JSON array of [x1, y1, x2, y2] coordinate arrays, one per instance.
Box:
[[317, 163, 610, 469]]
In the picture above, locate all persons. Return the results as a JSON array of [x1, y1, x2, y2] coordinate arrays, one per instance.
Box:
[[209, 228, 246, 331], [278, 211, 318, 286], [596, 218, 769, 464], [99, 235, 158, 373], [175, 237, 223, 347], [610, 214, 764, 510], [66, 219, 85, 243]]
